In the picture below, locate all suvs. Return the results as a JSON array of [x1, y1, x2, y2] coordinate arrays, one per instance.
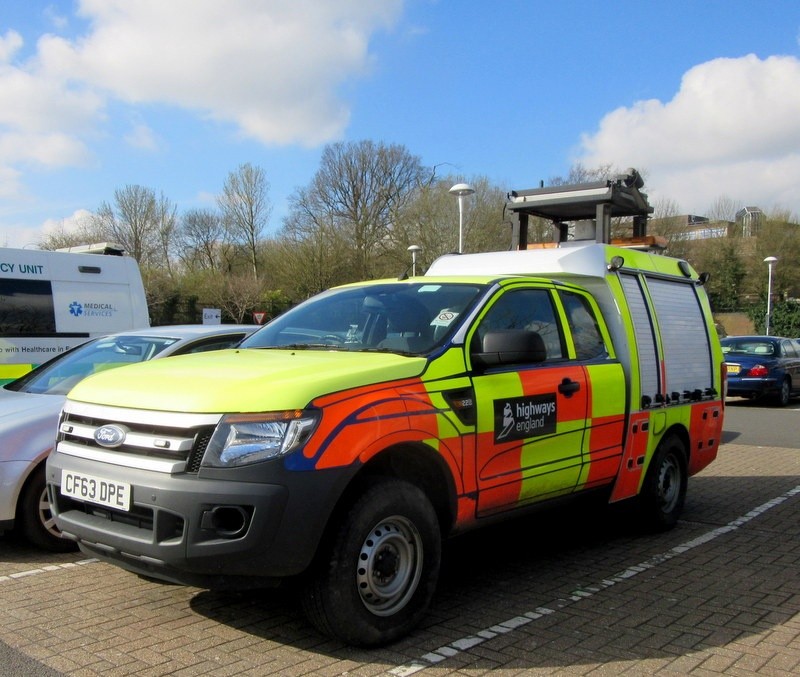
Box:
[[720, 335, 800, 407]]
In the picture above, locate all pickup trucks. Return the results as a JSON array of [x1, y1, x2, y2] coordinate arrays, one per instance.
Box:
[[46, 243, 730, 651]]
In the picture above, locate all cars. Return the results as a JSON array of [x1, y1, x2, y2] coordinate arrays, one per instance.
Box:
[[0, 321, 359, 554]]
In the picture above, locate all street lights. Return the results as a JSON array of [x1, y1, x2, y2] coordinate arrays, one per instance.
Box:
[[449, 182, 476, 254], [407, 244, 422, 276], [763, 256, 778, 336]]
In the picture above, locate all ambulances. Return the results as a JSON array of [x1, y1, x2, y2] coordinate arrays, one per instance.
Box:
[[0, 239, 152, 392]]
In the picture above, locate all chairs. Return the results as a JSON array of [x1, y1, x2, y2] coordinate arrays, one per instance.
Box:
[[377, 296, 431, 351]]
